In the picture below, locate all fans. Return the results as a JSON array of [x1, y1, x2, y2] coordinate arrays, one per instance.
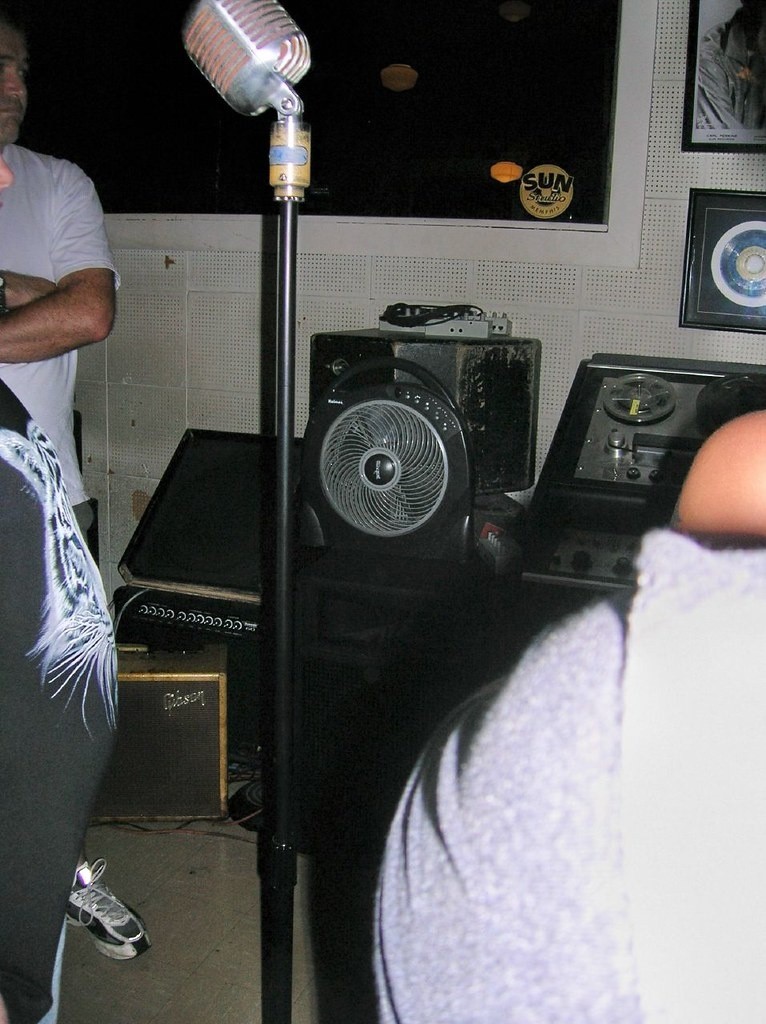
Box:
[[303, 357, 474, 579]]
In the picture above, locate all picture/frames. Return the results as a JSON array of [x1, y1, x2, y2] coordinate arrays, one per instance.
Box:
[[681, 0, 766, 153], [678, 186, 766, 335]]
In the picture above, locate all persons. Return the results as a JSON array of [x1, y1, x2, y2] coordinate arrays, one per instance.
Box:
[[0, 12, 152, 960], [0, 379, 118, 1024], [375, 411, 766, 1024]]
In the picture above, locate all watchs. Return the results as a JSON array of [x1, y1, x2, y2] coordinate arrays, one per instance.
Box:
[[0, 275, 6, 313]]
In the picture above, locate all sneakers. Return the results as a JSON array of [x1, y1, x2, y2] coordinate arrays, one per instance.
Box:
[[64, 858, 151, 959]]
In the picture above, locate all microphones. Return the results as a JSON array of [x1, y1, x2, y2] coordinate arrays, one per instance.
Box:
[[181, 0, 312, 117]]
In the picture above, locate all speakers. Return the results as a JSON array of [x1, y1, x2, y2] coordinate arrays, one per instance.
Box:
[[297, 573, 529, 857], [112, 584, 278, 774], [86, 647, 229, 824]]
